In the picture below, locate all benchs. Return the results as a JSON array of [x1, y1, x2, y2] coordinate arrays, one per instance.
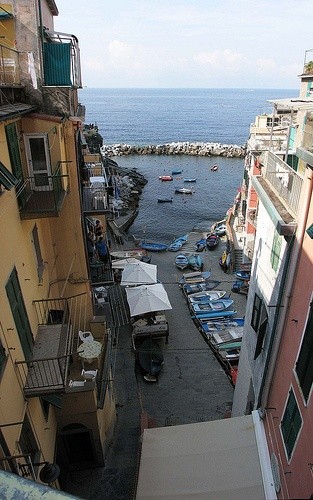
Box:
[[194, 304, 201, 310], [212, 334, 223, 343], [197, 284, 203, 291], [132, 322, 170, 345], [208, 303, 215, 310], [228, 330, 239, 339], [214, 324, 221, 329], [206, 292, 212, 300]]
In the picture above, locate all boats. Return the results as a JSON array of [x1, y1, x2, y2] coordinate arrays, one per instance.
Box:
[[172, 171, 182, 174], [211, 166, 217, 171], [200, 318, 245, 345], [196, 311, 238, 320], [189, 253, 204, 270], [158, 198, 173, 202], [175, 253, 188, 270], [207, 233, 219, 250], [184, 179, 197, 182], [175, 189, 193, 194], [194, 239, 207, 252], [184, 280, 220, 295], [169, 241, 183, 252], [192, 299, 235, 314], [188, 290, 227, 303], [178, 270, 212, 285], [229, 365, 238, 387], [138, 242, 169, 251], [175, 236, 188, 245], [159, 176, 173, 181], [213, 221, 226, 236], [218, 341, 241, 364]]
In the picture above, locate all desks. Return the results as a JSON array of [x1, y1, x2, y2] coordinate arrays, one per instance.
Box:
[[96, 286, 108, 298], [155, 314, 166, 323], [89, 177, 106, 186], [77, 341, 103, 364], [134, 318, 148, 328]]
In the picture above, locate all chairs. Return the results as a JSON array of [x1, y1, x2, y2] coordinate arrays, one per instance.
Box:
[[94, 294, 106, 310], [81, 369, 99, 381], [68, 380, 87, 388], [78, 330, 95, 343], [90, 181, 107, 210]]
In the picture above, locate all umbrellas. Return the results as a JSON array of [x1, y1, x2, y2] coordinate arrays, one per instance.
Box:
[[125, 283, 172, 317], [120, 263, 157, 286]]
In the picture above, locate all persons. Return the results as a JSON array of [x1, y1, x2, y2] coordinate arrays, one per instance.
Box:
[[96, 237, 109, 268], [94, 220, 104, 242], [88, 231, 95, 257]]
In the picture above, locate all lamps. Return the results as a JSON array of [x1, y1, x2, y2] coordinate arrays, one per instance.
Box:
[[20, 461, 60, 488]]
